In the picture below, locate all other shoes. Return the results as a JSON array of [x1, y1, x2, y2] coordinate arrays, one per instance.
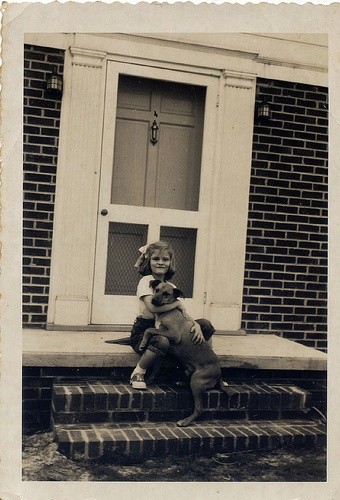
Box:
[[129, 369, 146, 388]]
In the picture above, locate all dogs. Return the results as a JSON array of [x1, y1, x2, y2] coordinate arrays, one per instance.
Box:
[[137, 278, 235, 428]]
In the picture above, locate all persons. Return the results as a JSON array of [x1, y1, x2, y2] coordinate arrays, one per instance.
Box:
[[129, 241, 216, 390]]
